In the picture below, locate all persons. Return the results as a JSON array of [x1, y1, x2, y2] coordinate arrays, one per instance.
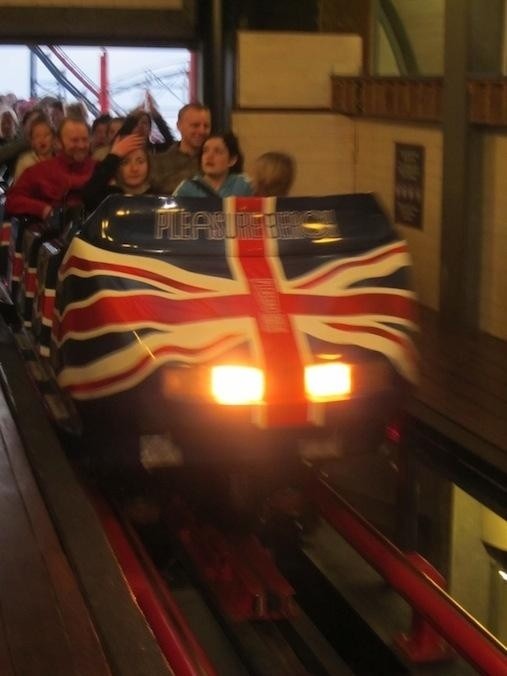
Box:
[[1, 93, 297, 247]]
